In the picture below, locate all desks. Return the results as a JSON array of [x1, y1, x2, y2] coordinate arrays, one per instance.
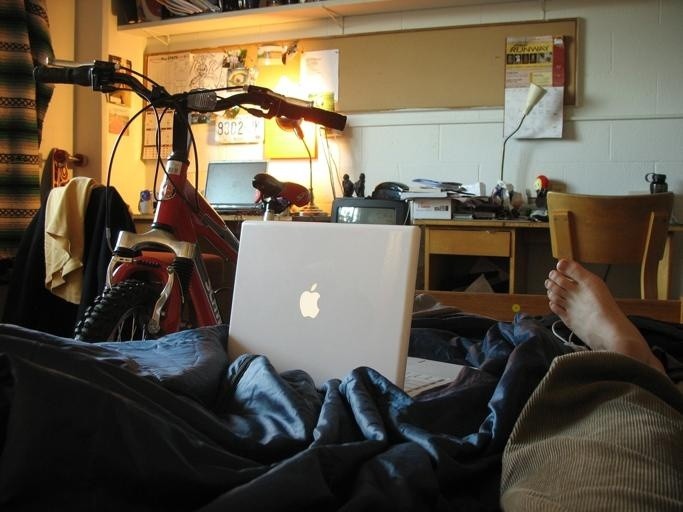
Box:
[[132, 212, 266, 236], [412, 216, 682, 303]]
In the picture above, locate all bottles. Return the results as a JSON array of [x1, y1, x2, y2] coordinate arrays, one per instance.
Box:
[[644, 172, 667, 195]]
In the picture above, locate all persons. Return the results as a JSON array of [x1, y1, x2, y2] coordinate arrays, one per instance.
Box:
[[342, 173, 354, 197], [354, 172, 366, 198], [496, 256, 681, 510]]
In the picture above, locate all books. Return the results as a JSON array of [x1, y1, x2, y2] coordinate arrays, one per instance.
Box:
[[399, 177, 504, 221], [116, 0, 321, 27]]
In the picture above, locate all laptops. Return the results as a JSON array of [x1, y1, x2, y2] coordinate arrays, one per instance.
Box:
[[226, 220, 483, 399]]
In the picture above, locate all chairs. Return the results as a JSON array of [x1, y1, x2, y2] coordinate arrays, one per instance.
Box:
[[546, 188, 676, 298]]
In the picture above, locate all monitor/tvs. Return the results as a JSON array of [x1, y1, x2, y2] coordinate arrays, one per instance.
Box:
[[204, 160, 269, 212], [330, 199, 409, 225]]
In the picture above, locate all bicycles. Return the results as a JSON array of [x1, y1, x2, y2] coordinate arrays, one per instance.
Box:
[[30, 59, 346, 343]]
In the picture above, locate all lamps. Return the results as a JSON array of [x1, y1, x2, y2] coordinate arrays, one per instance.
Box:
[[494, 82, 548, 206], [309, 89, 339, 204], [275, 101, 329, 223]]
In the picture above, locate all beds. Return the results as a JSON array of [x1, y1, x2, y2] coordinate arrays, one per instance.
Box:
[[1, 293, 680, 510]]
